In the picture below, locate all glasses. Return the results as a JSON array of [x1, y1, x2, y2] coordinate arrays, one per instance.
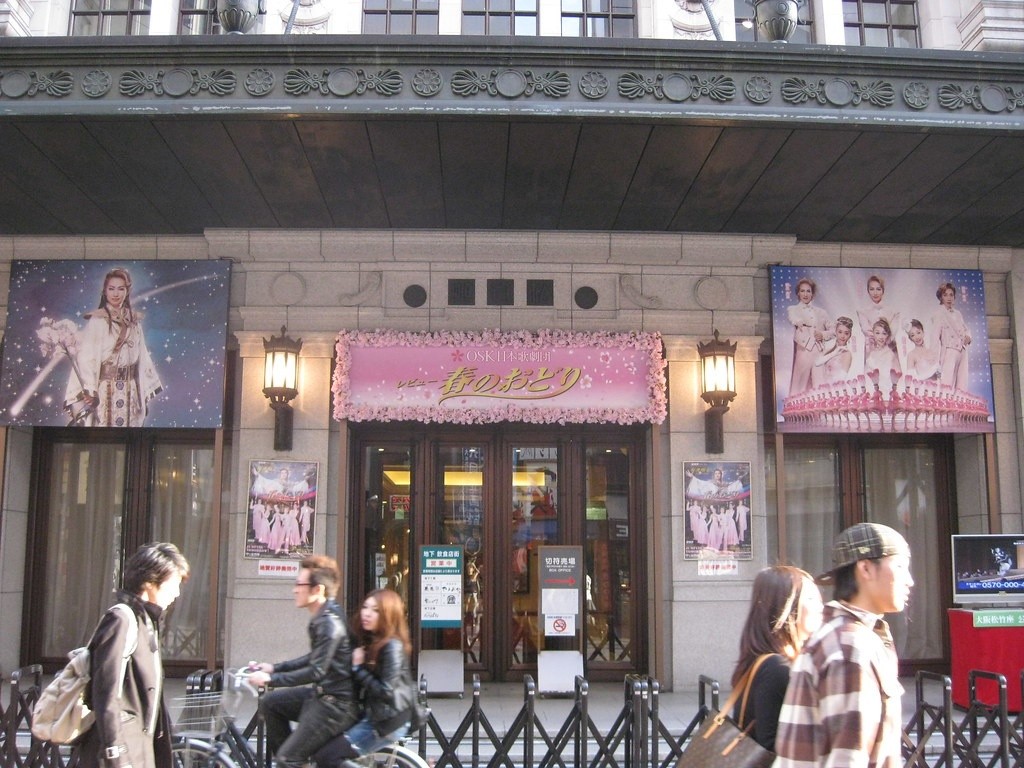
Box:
[[296, 581, 312, 586]]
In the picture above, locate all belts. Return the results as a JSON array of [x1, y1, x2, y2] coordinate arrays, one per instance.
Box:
[[98, 364, 136, 381]]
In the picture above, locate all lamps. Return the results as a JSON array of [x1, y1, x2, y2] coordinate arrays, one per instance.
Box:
[[262, 326, 305, 452], [695, 327, 740, 455]]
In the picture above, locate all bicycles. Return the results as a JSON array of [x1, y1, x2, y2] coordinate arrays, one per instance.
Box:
[[165, 661, 433, 768]]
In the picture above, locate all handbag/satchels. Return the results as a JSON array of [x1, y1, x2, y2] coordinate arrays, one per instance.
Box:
[[31, 604, 140, 744], [674, 651, 780, 768], [408, 681, 432, 734]]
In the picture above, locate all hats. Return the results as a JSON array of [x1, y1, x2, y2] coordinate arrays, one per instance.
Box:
[[814, 522, 911, 587]]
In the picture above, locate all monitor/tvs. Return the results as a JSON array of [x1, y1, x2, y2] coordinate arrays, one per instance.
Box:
[[951, 534, 1024, 604]]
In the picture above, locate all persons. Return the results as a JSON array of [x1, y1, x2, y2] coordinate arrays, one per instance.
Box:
[[76, 540, 190, 768], [246, 556, 360, 767], [770, 524, 916, 768], [63, 267, 163, 427], [786, 278, 991, 433], [728, 563, 826, 768], [686, 469, 749, 552], [315, 587, 419, 768], [991, 547, 1012, 576], [249, 466, 314, 555]]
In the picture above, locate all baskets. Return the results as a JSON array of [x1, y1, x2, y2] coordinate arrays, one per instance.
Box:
[[170, 689, 242, 739]]
